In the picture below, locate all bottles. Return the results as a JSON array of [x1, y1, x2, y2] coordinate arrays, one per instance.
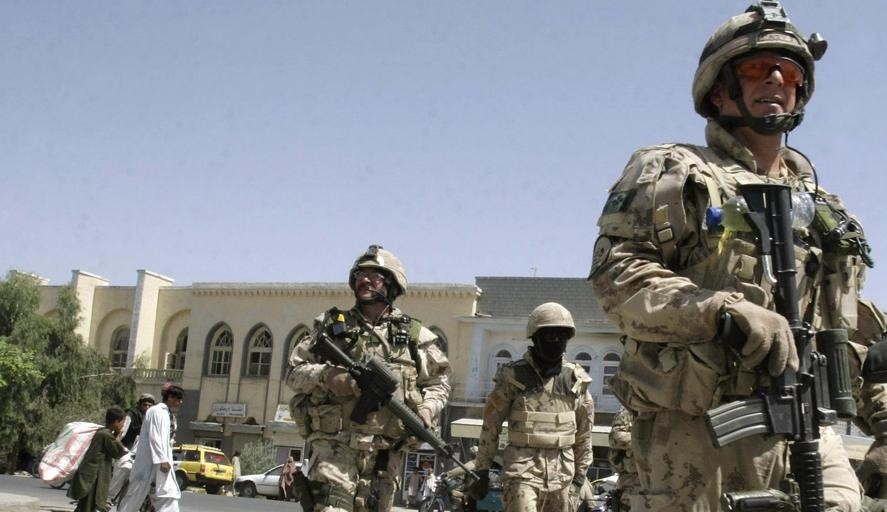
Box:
[[707, 193, 815, 232]]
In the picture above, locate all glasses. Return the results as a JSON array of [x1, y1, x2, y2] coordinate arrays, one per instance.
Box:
[[354, 271, 384, 281], [736, 55, 805, 85]]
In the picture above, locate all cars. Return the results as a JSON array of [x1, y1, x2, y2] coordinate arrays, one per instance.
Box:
[[234, 462, 305, 497], [589, 475, 617, 507]]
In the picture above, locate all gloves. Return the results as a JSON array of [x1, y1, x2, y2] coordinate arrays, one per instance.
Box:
[[326, 366, 361, 398], [408, 405, 432, 448], [469, 470, 489, 500], [724, 291, 800, 378]]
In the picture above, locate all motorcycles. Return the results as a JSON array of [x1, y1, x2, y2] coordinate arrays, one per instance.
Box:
[[417, 464, 506, 511]]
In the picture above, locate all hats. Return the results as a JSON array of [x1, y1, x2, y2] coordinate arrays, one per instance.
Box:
[[140, 393, 155, 403]]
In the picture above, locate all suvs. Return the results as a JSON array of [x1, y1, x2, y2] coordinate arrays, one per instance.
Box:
[[172, 444, 235, 493]]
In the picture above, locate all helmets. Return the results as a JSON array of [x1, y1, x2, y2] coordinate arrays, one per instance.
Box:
[[349, 245, 408, 296], [692, 0, 827, 117], [526, 302, 575, 338]]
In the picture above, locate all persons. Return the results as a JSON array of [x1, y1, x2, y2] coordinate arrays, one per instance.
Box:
[[405, 466, 437, 510], [585, 0, 887, 512], [283, 245, 455, 512], [71, 384, 183, 511], [226, 450, 241, 495], [279, 456, 310, 502], [443, 445, 479, 508], [468, 302, 602, 511], [606, 402, 643, 510]]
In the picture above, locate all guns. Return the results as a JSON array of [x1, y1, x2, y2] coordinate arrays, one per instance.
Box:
[[704, 186, 857, 512], [316, 331, 481, 482]]
